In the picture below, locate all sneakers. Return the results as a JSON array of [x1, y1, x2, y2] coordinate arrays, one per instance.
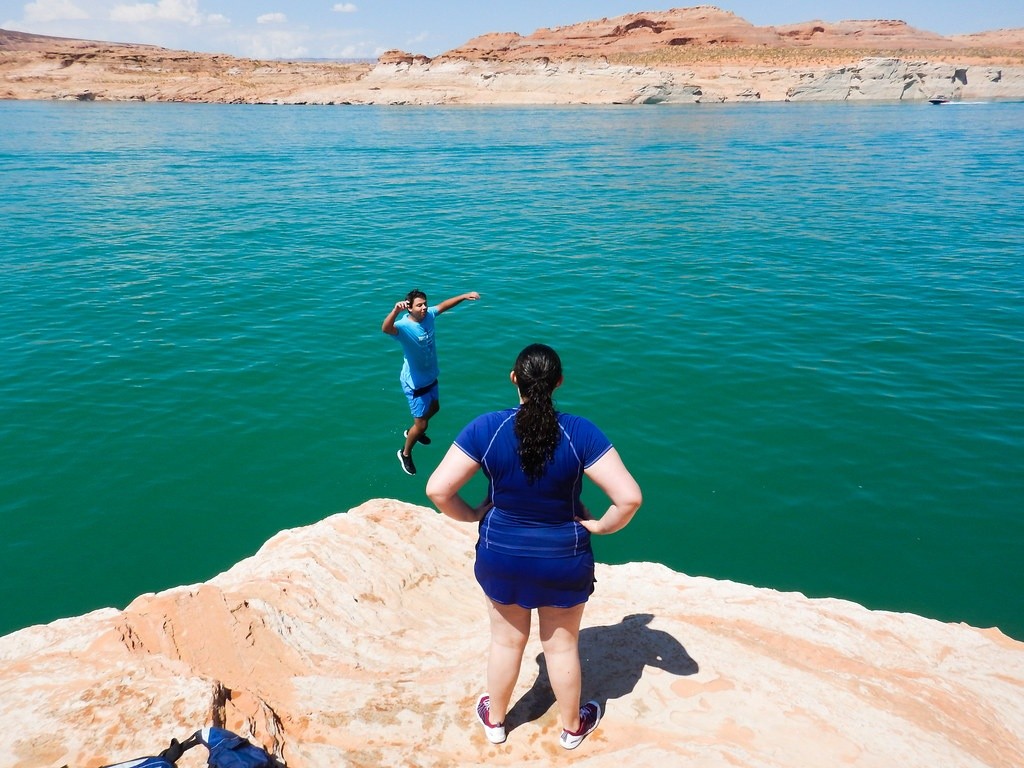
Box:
[[558, 702, 601, 749], [398, 448, 417, 475], [476, 692, 506, 743], [403, 430, 430, 445]]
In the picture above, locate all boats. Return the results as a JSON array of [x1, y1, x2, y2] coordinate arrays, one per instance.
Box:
[[928, 95, 950, 105]]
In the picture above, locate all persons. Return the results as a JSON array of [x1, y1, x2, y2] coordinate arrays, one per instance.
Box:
[[381, 288, 480, 476], [426, 343, 644, 749]]
